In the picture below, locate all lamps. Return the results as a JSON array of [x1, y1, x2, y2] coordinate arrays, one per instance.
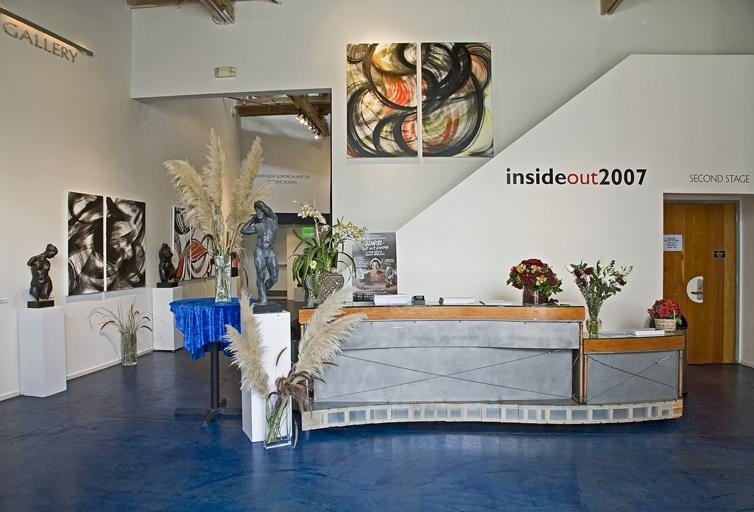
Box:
[[295, 104, 321, 142]]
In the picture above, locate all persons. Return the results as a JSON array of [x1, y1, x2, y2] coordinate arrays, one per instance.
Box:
[[364, 258, 386, 287], [240, 200, 279, 306], [27, 244, 58, 299], [157, 242, 176, 282]]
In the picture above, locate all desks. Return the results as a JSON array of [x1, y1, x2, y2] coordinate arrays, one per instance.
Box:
[[161, 297, 258, 436]]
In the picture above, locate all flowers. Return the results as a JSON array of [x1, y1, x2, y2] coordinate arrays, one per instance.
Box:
[[287, 190, 367, 300], [645, 297, 684, 327], [563, 253, 639, 333], [504, 257, 563, 299]]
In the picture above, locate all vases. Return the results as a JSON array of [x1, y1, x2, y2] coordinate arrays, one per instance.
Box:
[[584, 317, 603, 336], [209, 253, 234, 303], [303, 269, 345, 310], [260, 393, 294, 451], [118, 332, 139, 366], [652, 310, 679, 333], [521, 285, 549, 306]]
